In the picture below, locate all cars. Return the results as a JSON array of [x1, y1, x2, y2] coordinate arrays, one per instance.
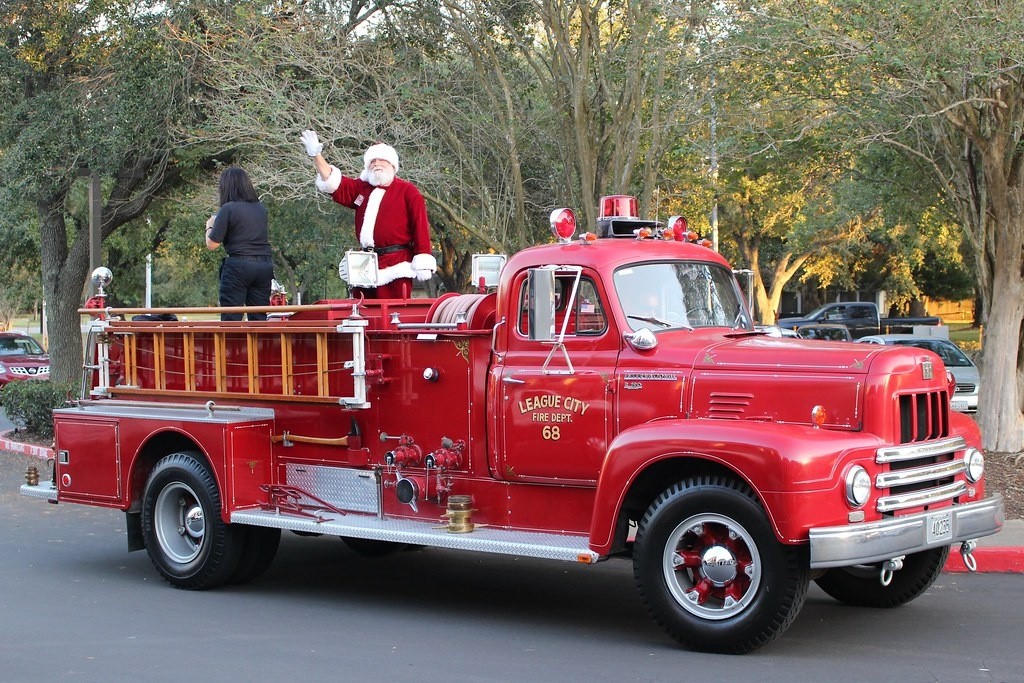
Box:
[[796, 322, 854, 341], [853, 333, 979, 414], [754, 324, 803, 340], [0, 333, 51, 408]]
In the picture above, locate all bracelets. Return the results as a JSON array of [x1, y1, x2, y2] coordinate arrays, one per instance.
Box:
[[205, 226, 214, 233]]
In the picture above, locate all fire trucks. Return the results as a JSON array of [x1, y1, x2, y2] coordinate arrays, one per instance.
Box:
[[16, 195, 1009, 657]]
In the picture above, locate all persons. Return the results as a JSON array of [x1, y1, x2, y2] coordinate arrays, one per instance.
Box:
[[205, 167, 273, 322], [299, 129, 438, 299]]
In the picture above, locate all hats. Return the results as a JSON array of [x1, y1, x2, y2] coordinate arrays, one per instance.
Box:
[[364, 143, 399, 176]]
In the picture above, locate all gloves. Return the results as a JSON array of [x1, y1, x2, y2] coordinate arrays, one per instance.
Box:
[[299, 130, 323, 156], [415, 269, 432, 282]]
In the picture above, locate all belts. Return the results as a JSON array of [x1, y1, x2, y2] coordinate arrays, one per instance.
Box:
[[362, 245, 408, 255], [229, 254, 271, 261]]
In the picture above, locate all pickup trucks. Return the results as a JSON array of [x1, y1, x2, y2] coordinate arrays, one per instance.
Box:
[[776, 299, 943, 341]]
